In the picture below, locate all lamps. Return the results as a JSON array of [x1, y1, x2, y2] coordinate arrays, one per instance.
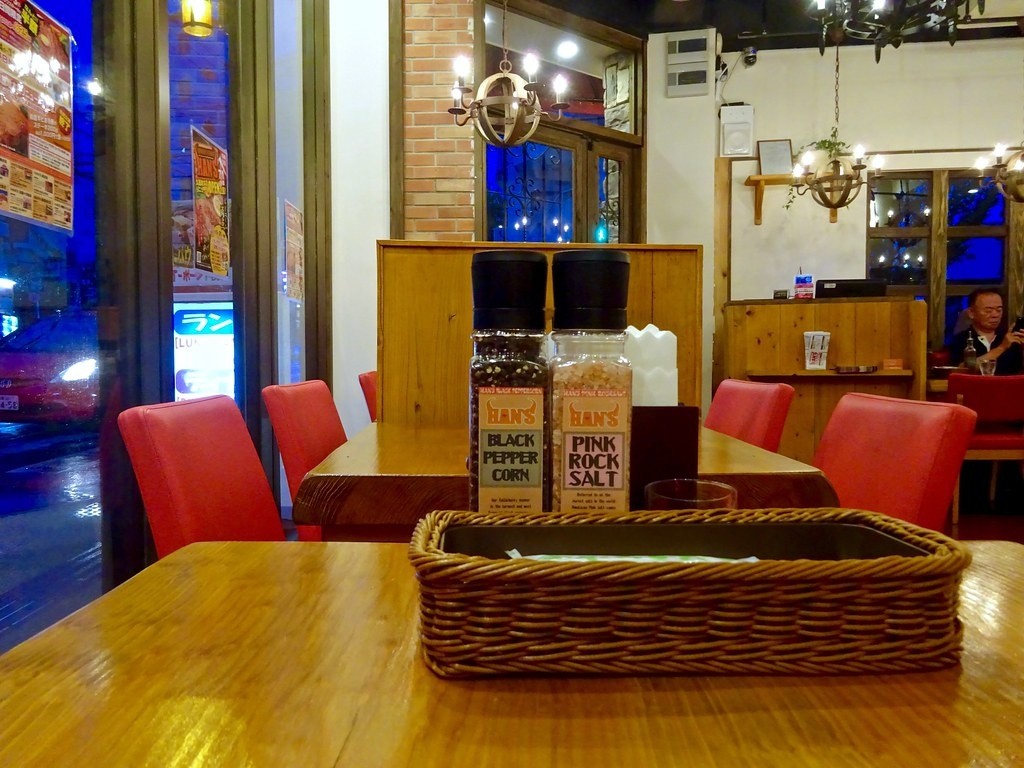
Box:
[[180, 0, 212, 35], [969, 139, 1023, 202], [809, 0, 986, 62], [860, 178, 935, 288], [782, 0, 881, 222], [448, 0, 569, 149]]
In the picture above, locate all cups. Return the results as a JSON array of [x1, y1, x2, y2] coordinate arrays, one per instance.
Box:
[[979, 359, 997, 376], [645, 478, 738, 510]]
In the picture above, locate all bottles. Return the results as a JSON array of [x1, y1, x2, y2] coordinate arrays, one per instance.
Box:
[[963, 331, 977, 375], [548, 249, 633, 512], [468, 251, 551, 513]]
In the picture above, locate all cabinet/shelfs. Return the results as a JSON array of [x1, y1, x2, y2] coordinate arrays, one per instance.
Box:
[[377, 235, 702, 476], [719, 294, 928, 465]]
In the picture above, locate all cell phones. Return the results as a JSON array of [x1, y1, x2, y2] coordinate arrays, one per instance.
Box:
[[1013, 317, 1024, 333]]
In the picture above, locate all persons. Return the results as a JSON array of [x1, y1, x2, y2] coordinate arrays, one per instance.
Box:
[[947, 288, 1024, 375]]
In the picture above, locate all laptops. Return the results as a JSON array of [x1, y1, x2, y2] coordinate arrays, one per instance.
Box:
[[815, 279, 887, 299]]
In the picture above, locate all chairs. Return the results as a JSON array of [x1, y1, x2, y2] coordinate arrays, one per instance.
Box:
[[359, 370, 378, 422], [118, 395, 288, 563], [262, 379, 348, 542], [705, 379, 794, 451], [927, 349, 1024, 525], [814, 391, 977, 535]]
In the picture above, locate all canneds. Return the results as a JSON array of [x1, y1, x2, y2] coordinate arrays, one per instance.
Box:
[[794, 274, 814, 299]]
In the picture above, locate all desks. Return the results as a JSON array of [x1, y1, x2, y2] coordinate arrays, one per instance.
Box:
[[293, 417, 841, 544], [0, 539, 1024, 768]]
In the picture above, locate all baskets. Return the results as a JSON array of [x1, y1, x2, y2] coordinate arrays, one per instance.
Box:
[[409, 510, 972, 676]]
